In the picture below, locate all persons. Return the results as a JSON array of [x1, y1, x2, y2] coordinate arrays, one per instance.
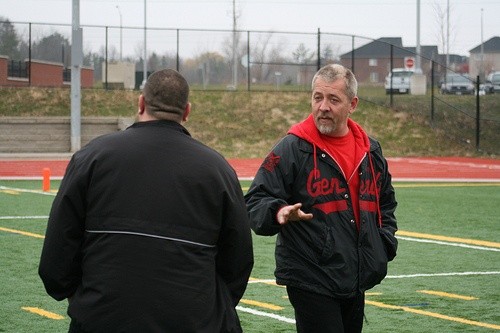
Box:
[[38, 68, 254, 333], [243, 63, 400, 333]]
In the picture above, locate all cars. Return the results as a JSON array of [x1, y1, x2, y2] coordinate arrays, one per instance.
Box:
[[485, 72, 500, 94], [384, 68, 423, 95], [439, 74, 476, 95]]
